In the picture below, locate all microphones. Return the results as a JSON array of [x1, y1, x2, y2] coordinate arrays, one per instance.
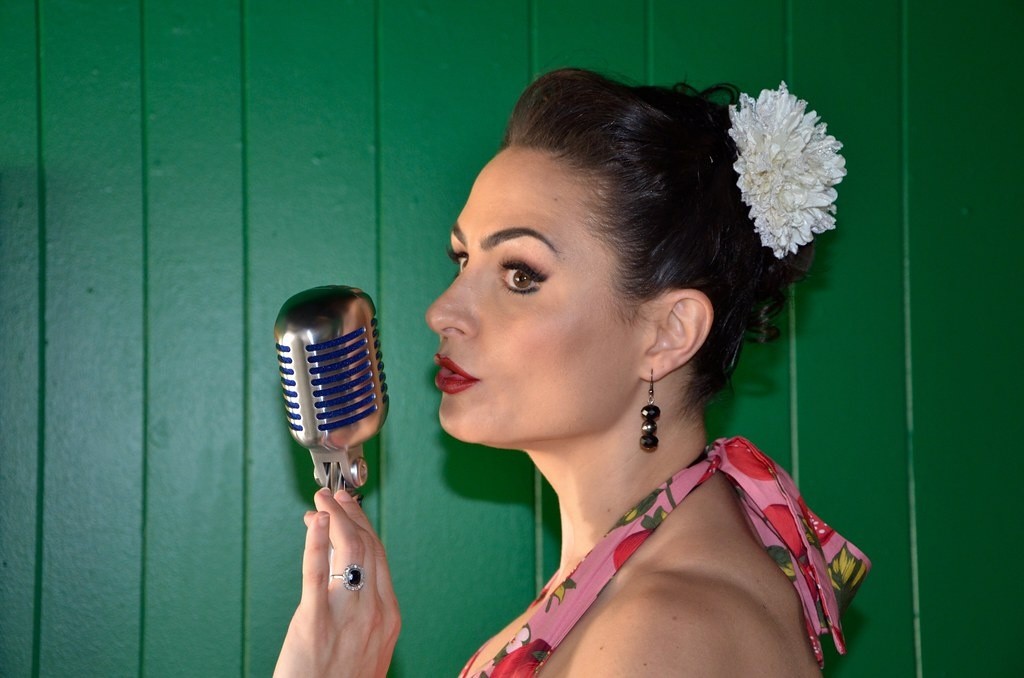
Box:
[[274, 285, 390, 495]]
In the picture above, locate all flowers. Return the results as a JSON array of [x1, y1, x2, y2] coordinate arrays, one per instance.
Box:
[[727, 79, 847, 259]]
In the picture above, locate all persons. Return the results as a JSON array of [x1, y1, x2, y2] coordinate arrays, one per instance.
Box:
[[272, 67, 871, 678]]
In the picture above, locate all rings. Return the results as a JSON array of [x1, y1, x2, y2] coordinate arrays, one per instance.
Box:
[[331, 564, 365, 591]]
